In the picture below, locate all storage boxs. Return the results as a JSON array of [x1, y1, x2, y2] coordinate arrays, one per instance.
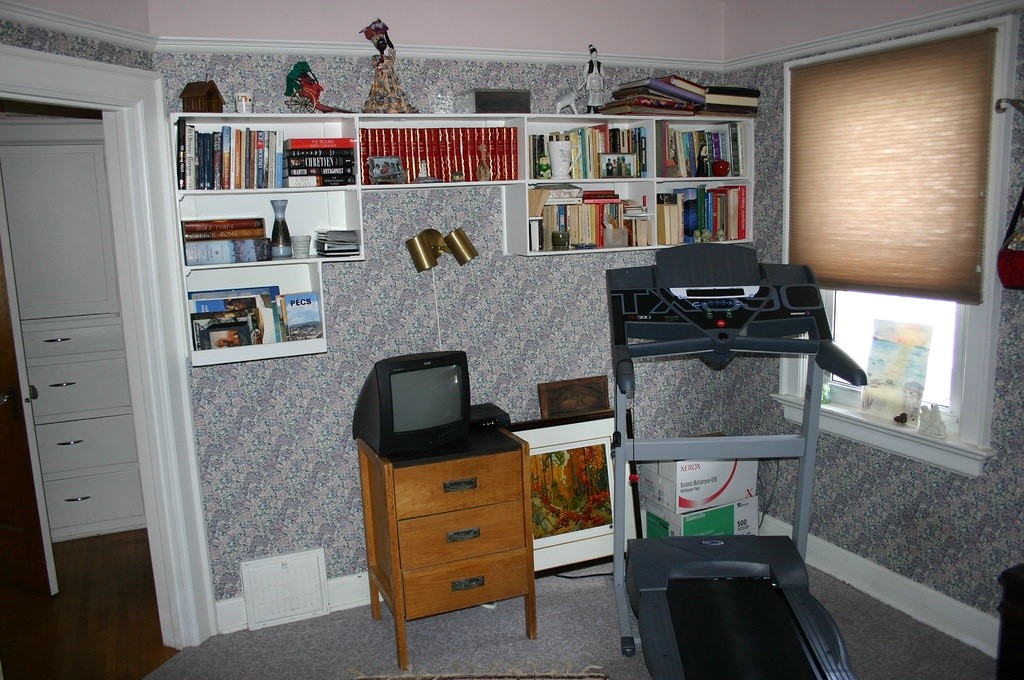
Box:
[[637, 430, 759, 541], [451, 89, 533, 114]]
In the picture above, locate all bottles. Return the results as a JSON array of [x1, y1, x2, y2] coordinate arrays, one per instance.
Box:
[[270, 200, 292, 257]]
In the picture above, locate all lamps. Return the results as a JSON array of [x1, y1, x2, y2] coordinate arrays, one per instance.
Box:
[[404, 227, 479, 277]]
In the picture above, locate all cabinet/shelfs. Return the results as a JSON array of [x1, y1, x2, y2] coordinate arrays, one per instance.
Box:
[[357, 428, 538, 671], [168, 111, 755, 366], [0, 117, 145, 543]]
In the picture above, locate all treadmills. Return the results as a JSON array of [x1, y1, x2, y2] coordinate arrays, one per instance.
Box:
[[606, 242, 868, 680]]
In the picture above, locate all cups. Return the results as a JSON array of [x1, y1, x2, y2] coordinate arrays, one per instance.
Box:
[[547, 141, 581, 179], [290, 236, 311, 259], [233, 93, 253, 114], [552, 232, 569, 251]]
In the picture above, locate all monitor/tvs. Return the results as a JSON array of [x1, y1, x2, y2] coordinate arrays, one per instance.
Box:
[[352, 351, 471, 461]]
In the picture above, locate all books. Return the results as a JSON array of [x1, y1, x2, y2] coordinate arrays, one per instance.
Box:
[[656, 186, 747, 245], [176, 119, 355, 191], [599, 75, 761, 116], [661, 121, 746, 177], [528, 183, 653, 252], [182, 218, 273, 266], [359, 127, 518, 185], [316, 230, 360, 257], [529, 123, 648, 179]]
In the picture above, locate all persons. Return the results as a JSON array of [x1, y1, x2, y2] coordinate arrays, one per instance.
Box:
[[381, 165, 387, 174], [389, 163, 396, 173], [612, 159, 617, 176], [386, 163, 390, 170], [697, 145, 709, 176], [617, 158, 622, 176], [584, 45, 606, 114], [621, 159, 627, 176], [373, 164, 381, 174], [606, 159, 612, 176], [717, 228, 726, 242], [694, 230, 701, 243], [702, 230, 711, 242], [362, 23, 420, 113]]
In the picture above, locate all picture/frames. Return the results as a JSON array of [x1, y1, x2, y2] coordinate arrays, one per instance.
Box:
[[597, 152, 636, 179], [367, 155, 407, 185], [509, 409, 643, 580], [538, 374, 612, 418]]
